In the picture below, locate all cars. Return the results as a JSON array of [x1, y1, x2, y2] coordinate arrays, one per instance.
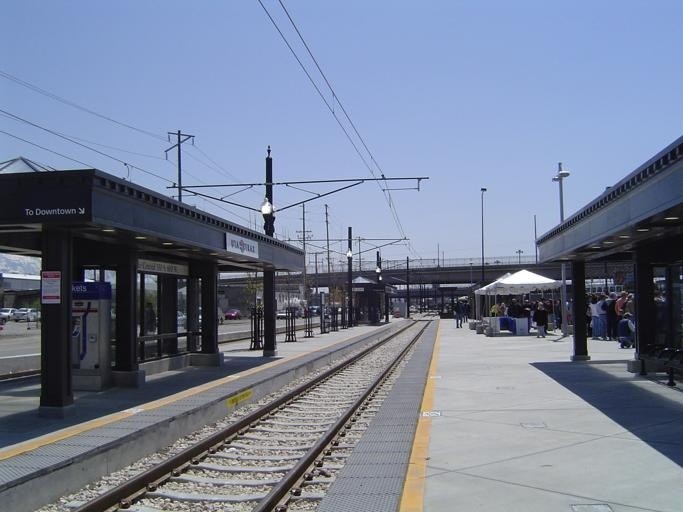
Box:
[[408, 303, 429, 314], [0, 319, 6, 331], [12, 307, 41, 322], [177, 301, 242, 330], [277, 303, 342, 321], [0, 306, 18, 321]]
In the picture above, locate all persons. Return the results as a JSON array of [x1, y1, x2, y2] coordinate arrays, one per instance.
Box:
[[490, 290, 635, 348], [454, 299, 471, 328], [143, 302, 157, 336]]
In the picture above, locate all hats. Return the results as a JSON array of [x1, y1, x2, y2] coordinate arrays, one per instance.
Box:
[[619, 291, 628, 295], [624, 313, 633, 318]]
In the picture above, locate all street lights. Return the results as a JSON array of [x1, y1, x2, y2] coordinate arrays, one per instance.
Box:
[[468, 262, 474, 286], [515, 249, 524, 264], [480, 188, 488, 280], [345, 248, 355, 327], [551, 160, 573, 339], [374, 266, 383, 319], [256, 194, 284, 358]]
